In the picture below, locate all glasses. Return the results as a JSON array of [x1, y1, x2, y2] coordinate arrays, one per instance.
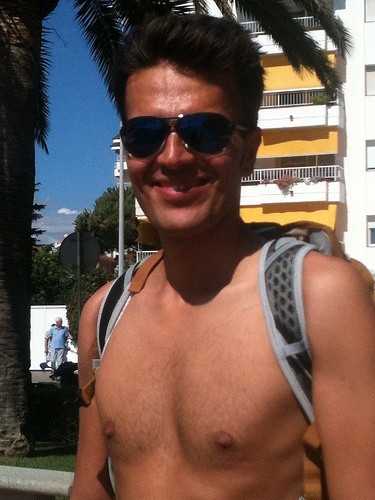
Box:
[[120, 113, 249, 159]]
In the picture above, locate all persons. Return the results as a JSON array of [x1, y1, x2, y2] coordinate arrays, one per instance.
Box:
[[45, 318, 74, 381], [68, 11, 374, 500]]
